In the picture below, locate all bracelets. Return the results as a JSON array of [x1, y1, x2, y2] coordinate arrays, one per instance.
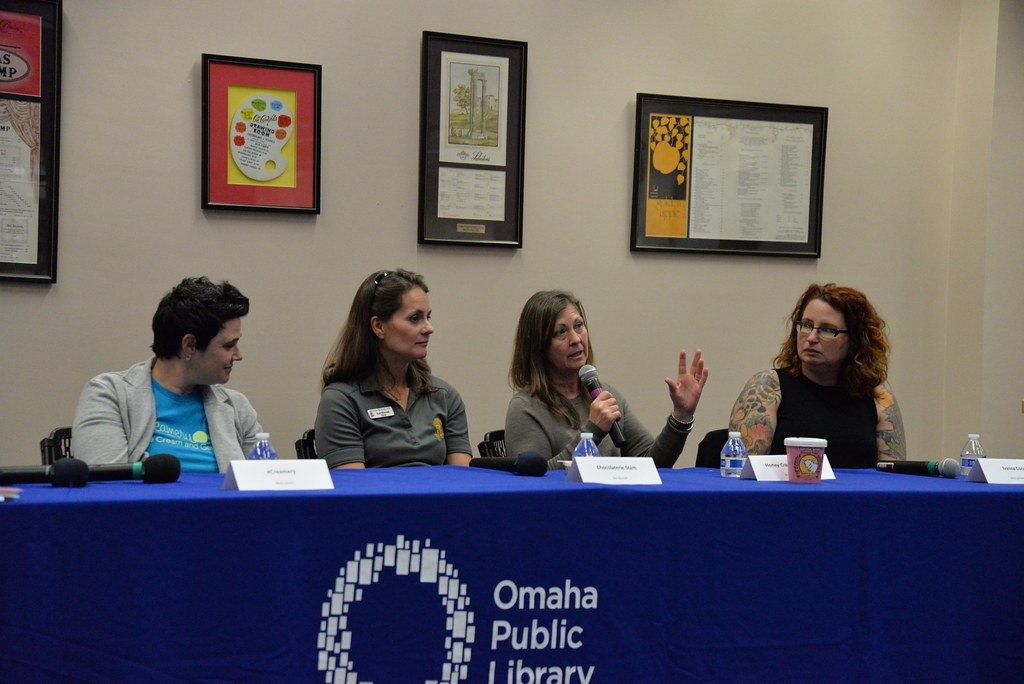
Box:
[[667, 412, 696, 431]]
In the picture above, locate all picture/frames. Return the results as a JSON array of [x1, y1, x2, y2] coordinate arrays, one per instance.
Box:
[[630, 92, 829, 258], [201, 53, 323, 215], [417, 30, 529, 249], [0, 0, 62, 283]]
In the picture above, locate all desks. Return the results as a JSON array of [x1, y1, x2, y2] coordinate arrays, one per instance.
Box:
[[0, 465, 1024, 684]]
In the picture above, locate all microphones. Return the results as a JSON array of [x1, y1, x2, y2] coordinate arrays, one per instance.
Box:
[[578, 364, 627, 448], [876, 458, 960, 477], [87, 453, 181, 483], [0, 458, 88, 487], [470, 451, 548, 477]]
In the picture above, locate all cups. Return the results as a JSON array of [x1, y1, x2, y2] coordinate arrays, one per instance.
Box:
[[784, 436, 828, 484]]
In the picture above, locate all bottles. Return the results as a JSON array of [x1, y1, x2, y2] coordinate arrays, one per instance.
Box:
[[720, 432, 748, 478], [571, 433, 600, 460], [960, 434, 986, 482], [246, 432, 279, 460]]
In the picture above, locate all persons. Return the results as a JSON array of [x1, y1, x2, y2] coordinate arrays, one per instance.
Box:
[[729, 283, 908, 468], [72, 276, 272, 474], [315, 267, 473, 470], [504, 288, 709, 471]]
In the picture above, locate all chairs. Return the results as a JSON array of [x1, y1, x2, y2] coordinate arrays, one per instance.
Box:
[[295, 429, 318, 460], [695, 429, 730, 469], [478, 430, 506, 458], [40, 426, 72, 467]]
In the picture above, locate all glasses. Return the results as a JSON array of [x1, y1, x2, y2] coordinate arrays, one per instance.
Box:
[[373, 271, 393, 300], [794, 321, 847, 339]]
